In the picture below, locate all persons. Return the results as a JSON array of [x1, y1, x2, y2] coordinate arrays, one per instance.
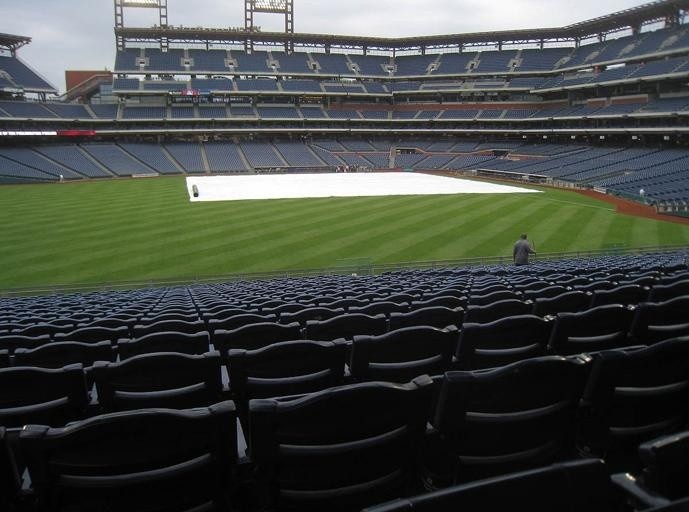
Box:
[[513, 233, 536, 266]]
